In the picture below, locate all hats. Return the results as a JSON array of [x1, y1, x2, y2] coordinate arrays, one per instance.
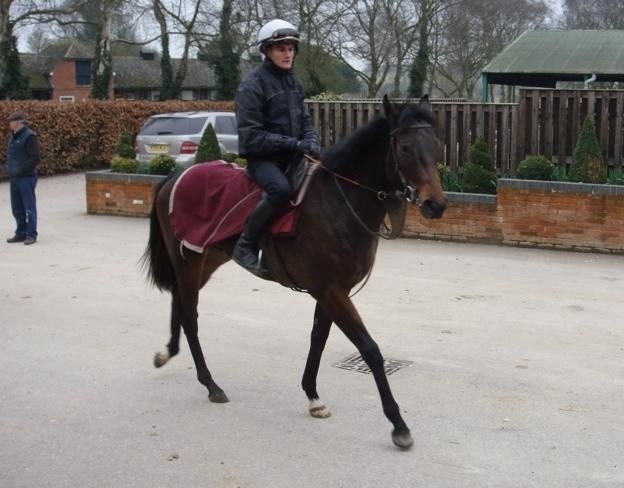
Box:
[[6, 112, 24, 122]]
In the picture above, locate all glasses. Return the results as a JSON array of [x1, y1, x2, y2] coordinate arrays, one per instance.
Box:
[[271, 28, 299, 38]]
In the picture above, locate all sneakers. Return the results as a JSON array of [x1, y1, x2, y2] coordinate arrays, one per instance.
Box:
[[6, 235, 25, 242], [23, 236, 35, 245]]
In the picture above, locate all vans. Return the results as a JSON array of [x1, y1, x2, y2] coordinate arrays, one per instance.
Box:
[[133, 109, 242, 171]]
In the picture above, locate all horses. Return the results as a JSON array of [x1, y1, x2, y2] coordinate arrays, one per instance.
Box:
[[138, 93, 450, 451]]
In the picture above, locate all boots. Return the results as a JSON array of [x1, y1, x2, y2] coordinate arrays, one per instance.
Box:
[[230, 199, 275, 276]]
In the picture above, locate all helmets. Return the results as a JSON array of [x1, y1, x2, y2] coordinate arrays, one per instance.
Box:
[[256, 19, 299, 55]]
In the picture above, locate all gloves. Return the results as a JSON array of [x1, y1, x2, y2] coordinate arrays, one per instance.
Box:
[[297, 132, 325, 157]]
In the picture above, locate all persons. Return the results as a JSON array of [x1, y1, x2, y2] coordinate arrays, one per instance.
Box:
[[3, 112, 42, 244], [231, 18, 326, 279]]
